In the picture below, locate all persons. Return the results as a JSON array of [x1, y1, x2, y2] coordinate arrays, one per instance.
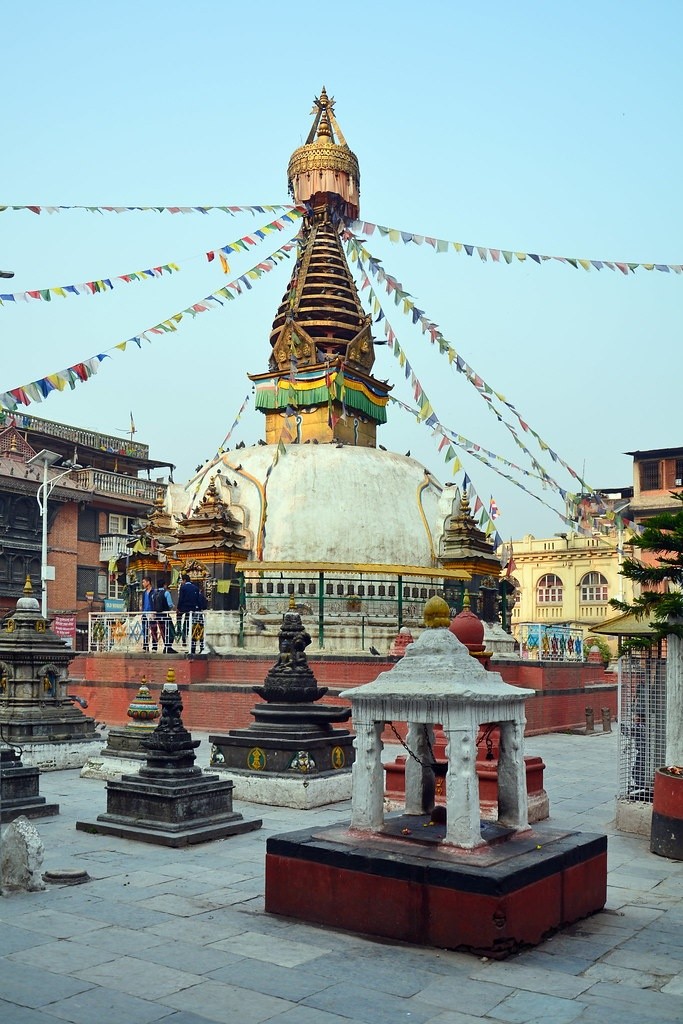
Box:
[[141, 574, 209, 654]]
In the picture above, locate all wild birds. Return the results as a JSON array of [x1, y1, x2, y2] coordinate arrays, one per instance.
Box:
[[369, 646, 380, 656]]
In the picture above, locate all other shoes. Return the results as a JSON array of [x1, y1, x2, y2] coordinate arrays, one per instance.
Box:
[[151, 646, 158, 653], [185, 648, 196, 654], [163, 647, 178, 654], [138, 650, 151, 654]]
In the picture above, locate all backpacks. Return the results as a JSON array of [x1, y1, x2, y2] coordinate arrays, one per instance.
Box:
[[197, 586, 208, 610], [153, 589, 166, 611]]
